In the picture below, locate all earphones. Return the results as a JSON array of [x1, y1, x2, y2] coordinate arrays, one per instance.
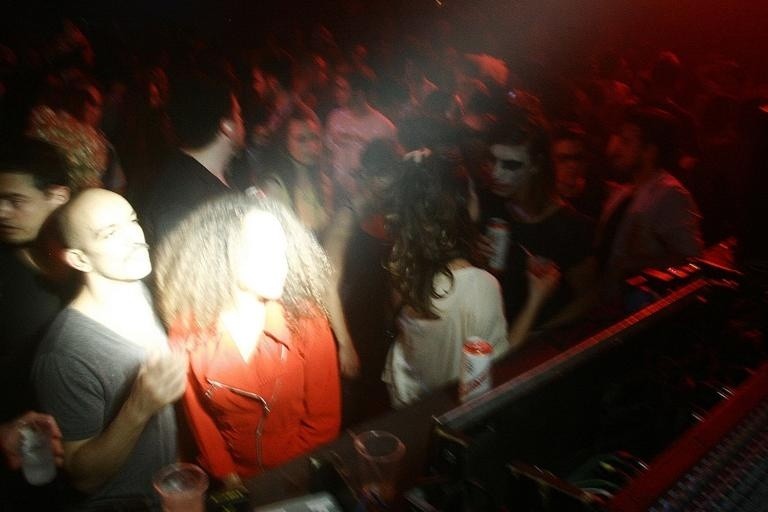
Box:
[[223, 123, 233, 131]]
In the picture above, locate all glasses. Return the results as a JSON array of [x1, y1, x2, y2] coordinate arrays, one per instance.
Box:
[[486, 150, 526, 171]]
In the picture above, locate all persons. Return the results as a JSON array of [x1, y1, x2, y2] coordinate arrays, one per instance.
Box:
[[0, 0, 768, 512]]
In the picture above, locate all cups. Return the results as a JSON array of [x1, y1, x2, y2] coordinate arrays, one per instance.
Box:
[[355, 430, 404, 507], [12, 419, 58, 486], [153, 462, 209, 512]]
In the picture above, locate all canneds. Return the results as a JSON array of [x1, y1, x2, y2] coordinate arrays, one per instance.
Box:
[[485, 216, 510, 277], [244, 184, 267, 200], [458, 336, 493, 403]]
